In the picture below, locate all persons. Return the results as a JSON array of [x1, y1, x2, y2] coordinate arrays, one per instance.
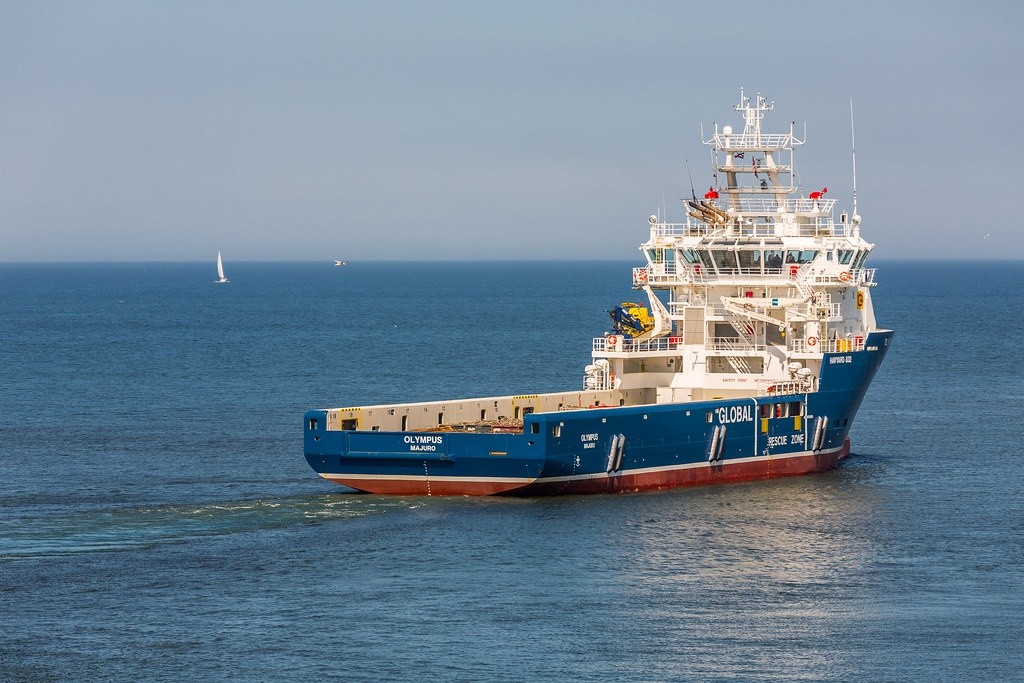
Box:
[[721, 251, 795, 268]]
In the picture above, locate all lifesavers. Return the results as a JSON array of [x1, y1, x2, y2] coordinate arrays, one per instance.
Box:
[[840, 271, 850, 281], [608, 335, 617, 345], [808, 336, 817, 346], [639, 270, 647, 280]]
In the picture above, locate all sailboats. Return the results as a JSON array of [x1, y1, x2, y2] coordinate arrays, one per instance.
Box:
[[214, 251, 230, 283]]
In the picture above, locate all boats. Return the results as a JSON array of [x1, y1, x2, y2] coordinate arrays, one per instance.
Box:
[[333, 260, 346, 266], [303, 85, 892, 497]]
